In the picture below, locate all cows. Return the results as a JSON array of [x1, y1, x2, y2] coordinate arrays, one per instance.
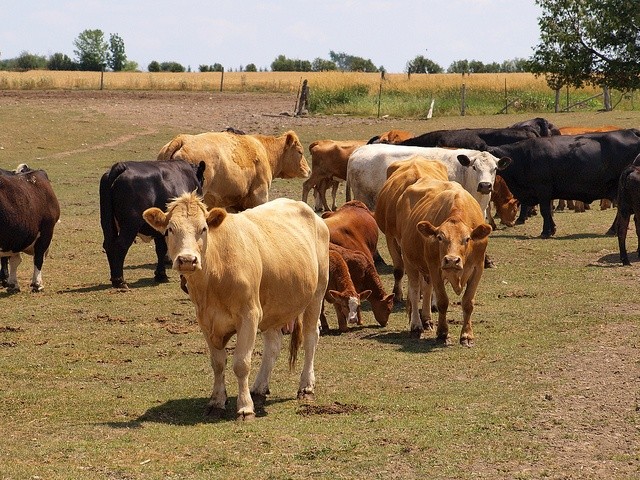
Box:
[[614, 164, 640, 266], [99, 158, 206, 290], [328, 244, 393, 329], [319, 199, 396, 328], [374, 156, 457, 313], [489, 175, 523, 231], [398, 126, 541, 228], [141, 186, 331, 422], [512, 117, 563, 135], [0, 163, 60, 294], [368, 130, 413, 143], [389, 176, 492, 349], [345, 142, 512, 225], [282, 247, 372, 335], [302, 139, 371, 213], [156, 129, 313, 214], [485, 128, 640, 239]]
